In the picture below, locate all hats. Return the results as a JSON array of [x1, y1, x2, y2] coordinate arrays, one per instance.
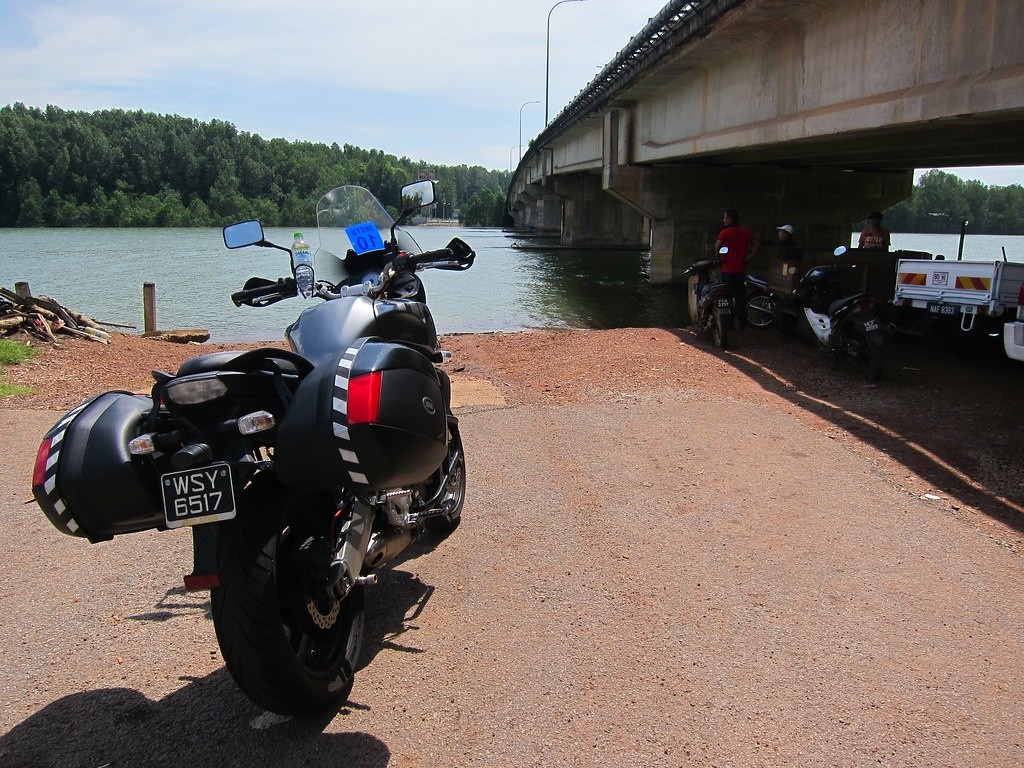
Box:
[[775, 224, 794, 236], [867, 212, 882, 220]]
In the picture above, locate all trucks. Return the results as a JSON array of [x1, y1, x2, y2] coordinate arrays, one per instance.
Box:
[[889, 258, 1024, 360]]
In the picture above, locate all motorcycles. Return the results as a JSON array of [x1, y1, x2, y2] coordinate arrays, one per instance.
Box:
[[31, 179, 477, 716], [744, 245, 826, 329], [682, 246, 737, 350], [788, 245, 877, 383]]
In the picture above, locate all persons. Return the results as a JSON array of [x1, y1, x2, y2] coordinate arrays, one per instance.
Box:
[[860, 213, 890, 252], [776, 225, 801, 260], [715, 208, 772, 330]]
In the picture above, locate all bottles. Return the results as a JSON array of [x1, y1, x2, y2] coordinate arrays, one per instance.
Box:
[[290, 232, 318, 298]]
